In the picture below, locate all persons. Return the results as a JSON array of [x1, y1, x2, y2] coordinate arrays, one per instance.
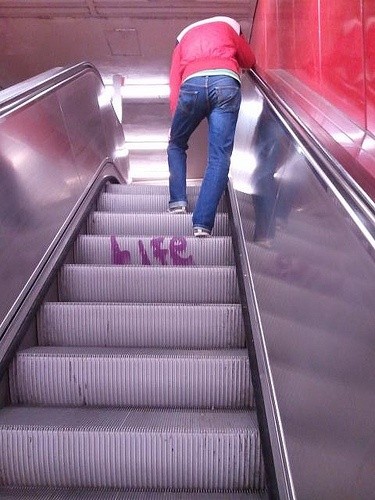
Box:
[[168, 16, 256, 236]]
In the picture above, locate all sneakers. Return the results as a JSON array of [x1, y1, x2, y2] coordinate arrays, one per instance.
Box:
[[166, 206, 187, 214], [193, 228, 211, 237]]
